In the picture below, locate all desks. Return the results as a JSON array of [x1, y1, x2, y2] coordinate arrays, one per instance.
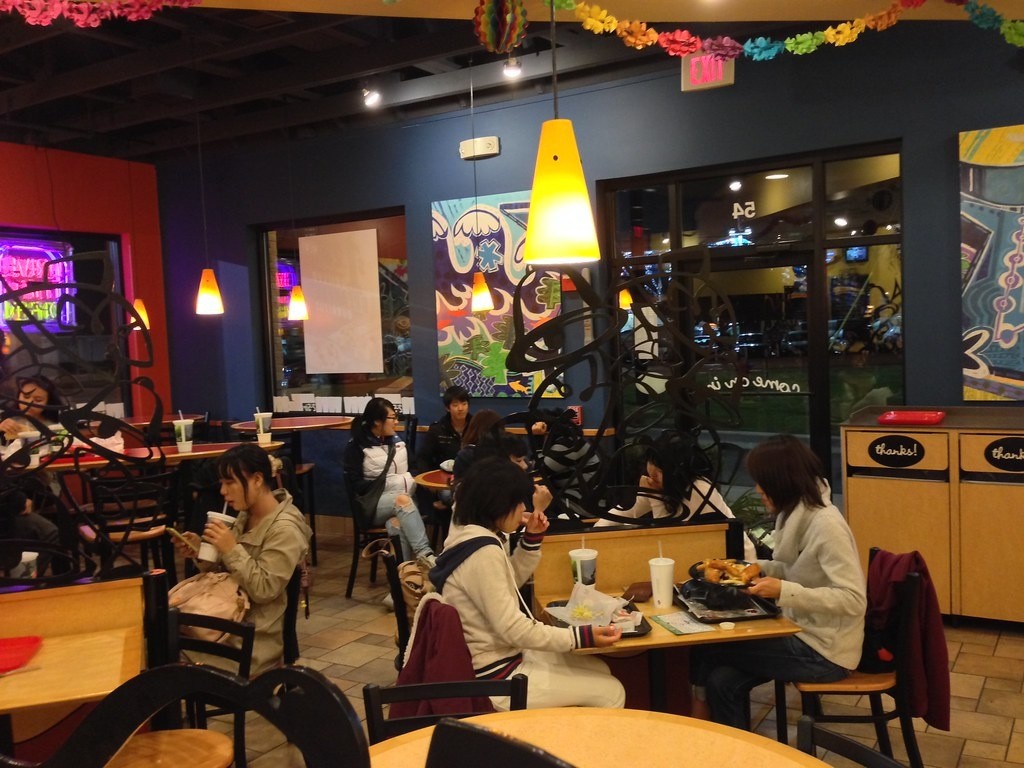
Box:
[[368, 706, 835, 768], [415, 469, 454, 489], [231, 416, 355, 464], [535, 581, 802, 714], [120, 414, 206, 424], [7, 442, 285, 472], [0, 625, 143, 768]]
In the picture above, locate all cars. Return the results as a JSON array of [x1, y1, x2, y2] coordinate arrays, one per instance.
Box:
[[693, 320, 849, 358]]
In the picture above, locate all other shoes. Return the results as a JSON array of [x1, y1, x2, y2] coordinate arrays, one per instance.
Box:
[[383, 592, 395, 612], [427, 555, 437, 565]]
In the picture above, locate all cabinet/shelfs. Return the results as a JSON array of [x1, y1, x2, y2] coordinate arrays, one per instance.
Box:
[[840, 406, 1023, 628]]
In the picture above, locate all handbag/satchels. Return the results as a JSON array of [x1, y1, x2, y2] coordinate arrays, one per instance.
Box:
[[354, 477, 387, 528], [168, 572, 250, 645]]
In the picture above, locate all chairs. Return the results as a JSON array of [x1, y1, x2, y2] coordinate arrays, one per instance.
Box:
[[0, 422, 951, 768]]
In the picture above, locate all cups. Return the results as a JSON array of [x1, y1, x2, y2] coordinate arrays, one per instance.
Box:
[[569, 549, 597, 590], [649, 558, 675, 608], [254, 413, 274, 443], [19, 431, 40, 466], [48, 424, 69, 451], [197, 511, 236, 564], [173, 419, 194, 453], [557, 512, 580, 520], [11, 552, 38, 579]]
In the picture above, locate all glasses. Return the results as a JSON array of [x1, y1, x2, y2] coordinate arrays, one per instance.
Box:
[[386, 414, 400, 420]]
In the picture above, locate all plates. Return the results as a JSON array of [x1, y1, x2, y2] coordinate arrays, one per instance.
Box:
[[688, 558, 766, 587]]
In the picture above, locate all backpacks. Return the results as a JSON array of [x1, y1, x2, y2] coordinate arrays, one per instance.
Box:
[[394, 559, 436, 648]]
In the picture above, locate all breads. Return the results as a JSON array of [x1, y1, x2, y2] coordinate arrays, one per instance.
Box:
[[697, 558, 763, 585]]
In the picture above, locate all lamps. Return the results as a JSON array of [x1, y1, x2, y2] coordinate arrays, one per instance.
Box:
[[468, 50, 494, 313], [287, 128, 309, 321], [127, 140, 150, 330], [523, 1, 600, 266], [196, 111, 225, 315]]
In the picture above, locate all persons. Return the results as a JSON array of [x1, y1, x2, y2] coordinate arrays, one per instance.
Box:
[[415, 384, 552, 535], [170, 443, 314, 676], [344, 398, 438, 609], [428, 456, 625, 712], [532, 408, 602, 500], [0, 375, 65, 576], [691, 435, 867, 732], [593, 431, 757, 560]]
